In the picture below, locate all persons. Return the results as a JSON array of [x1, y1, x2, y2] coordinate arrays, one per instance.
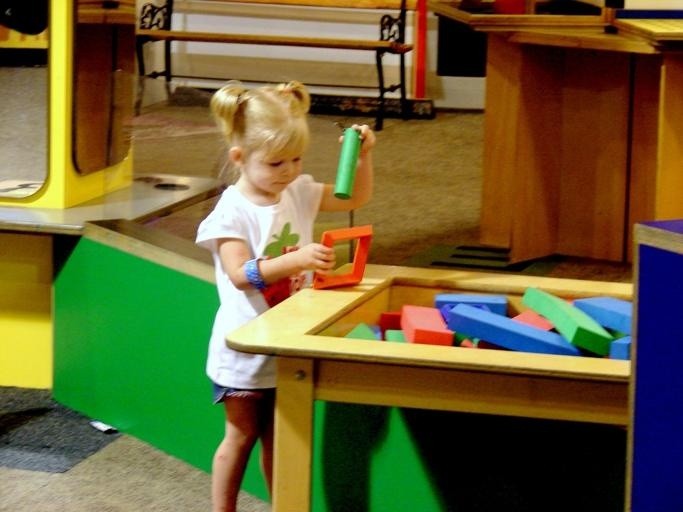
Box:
[[195, 79, 375, 512]]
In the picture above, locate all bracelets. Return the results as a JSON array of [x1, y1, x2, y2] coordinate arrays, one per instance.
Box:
[[243, 259, 267, 290]]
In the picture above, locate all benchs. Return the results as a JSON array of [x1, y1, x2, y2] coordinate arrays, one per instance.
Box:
[[131, 0, 435, 139]]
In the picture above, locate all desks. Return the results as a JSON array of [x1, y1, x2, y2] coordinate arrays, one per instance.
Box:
[[219, 255, 633, 512], [0, 174, 238, 400], [453, 1, 681, 266]]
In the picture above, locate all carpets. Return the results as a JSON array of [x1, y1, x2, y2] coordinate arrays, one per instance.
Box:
[[0, 383, 125, 475]]
